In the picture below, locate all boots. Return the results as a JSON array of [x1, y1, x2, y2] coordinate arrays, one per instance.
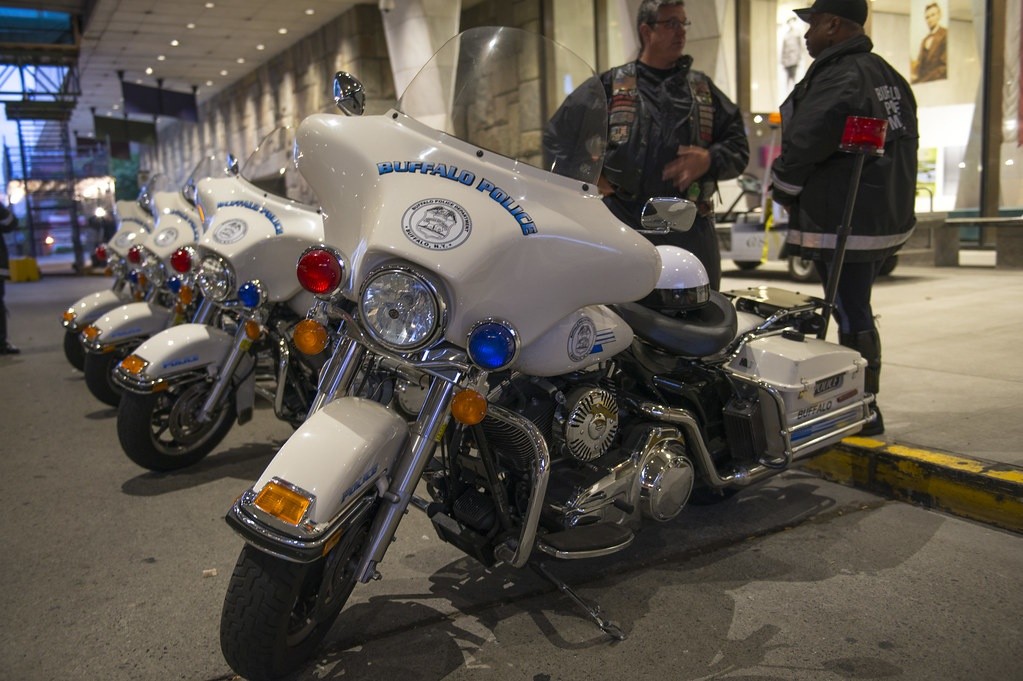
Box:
[[836, 327, 884, 436]]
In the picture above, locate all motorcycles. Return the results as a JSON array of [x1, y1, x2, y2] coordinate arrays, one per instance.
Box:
[[59, 125, 395, 471], [217, 24, 876, 680]]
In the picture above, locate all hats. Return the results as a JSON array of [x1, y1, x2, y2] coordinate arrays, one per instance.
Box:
[[792, 0, 868, 27]]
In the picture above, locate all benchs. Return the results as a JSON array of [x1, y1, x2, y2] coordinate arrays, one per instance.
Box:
[[931, 216, 1023, 268]]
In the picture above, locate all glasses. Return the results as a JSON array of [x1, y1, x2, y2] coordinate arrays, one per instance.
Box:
[[649, 16, 692, 31]]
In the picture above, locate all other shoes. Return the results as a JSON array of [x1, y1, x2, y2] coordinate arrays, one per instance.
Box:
[[0, 340, 20, 354]]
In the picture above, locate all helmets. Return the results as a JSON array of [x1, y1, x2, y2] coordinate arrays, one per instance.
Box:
[[636, 244, 710, 314]]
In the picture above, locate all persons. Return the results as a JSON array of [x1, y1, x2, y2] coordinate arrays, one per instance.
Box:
[[0, 202, 20, 354], [781, 17, 802, 88], [543, 0, 750, 291], [917, 3, 946, 82], [771, 0, 918, 434]]
